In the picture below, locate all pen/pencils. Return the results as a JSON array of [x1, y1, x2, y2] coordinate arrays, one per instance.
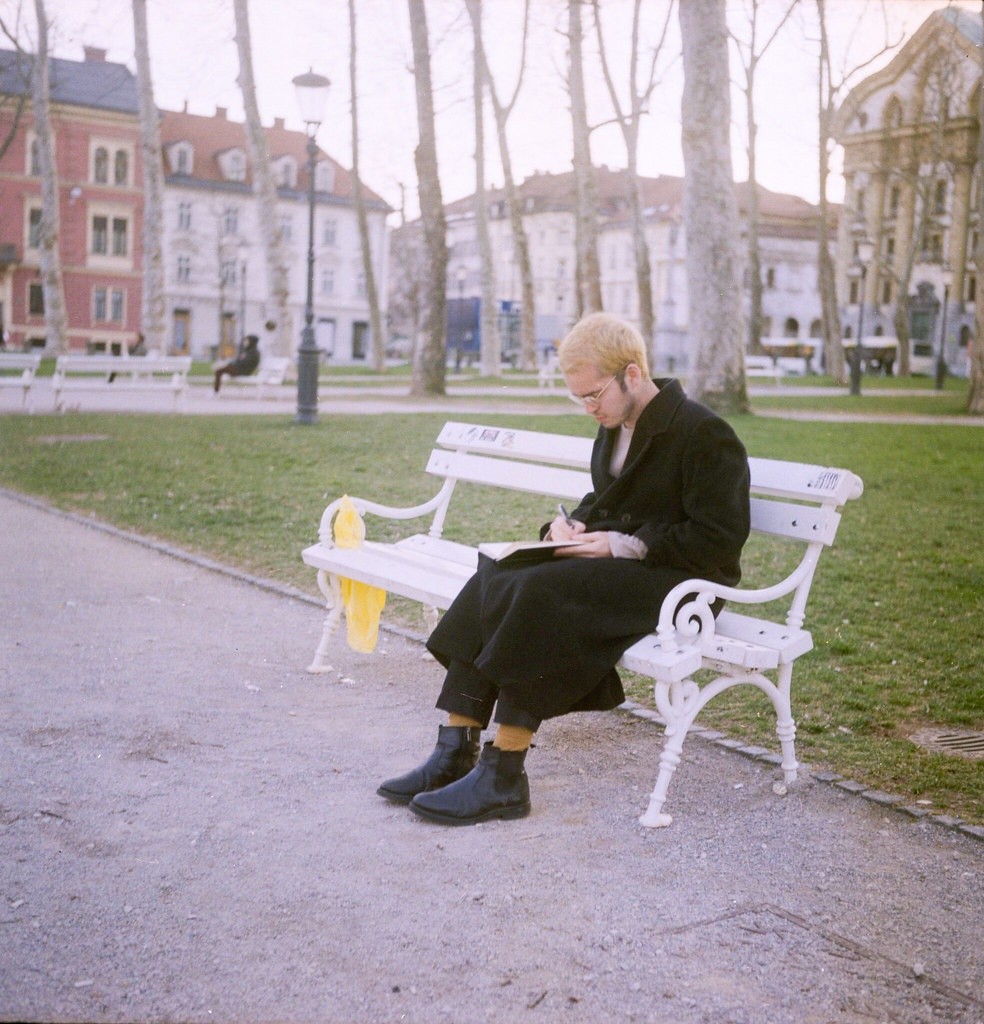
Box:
[[558, 503, 576, 530]]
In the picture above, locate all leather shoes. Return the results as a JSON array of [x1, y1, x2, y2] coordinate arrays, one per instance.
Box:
[[378, 723, 481, 804], [414, 743, 532, 822]]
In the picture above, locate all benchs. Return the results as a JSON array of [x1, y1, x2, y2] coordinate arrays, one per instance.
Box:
[[298, 418, 866, 835], [223, 357, 292, 404], [53, 353, 195, 419], [535, 356, 564, 394], [742, 354, 787, 387], [0, 352, 44, 416]]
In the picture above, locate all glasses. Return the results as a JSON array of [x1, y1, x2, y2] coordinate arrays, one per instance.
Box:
[[570, 361, 629, 407]]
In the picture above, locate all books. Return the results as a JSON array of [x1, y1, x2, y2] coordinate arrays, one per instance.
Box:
[[478, 537, 589, 565]]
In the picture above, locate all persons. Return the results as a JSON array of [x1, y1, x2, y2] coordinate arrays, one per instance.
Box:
[[212, 334, 264, 396], [378, 313, 751, 826]]
